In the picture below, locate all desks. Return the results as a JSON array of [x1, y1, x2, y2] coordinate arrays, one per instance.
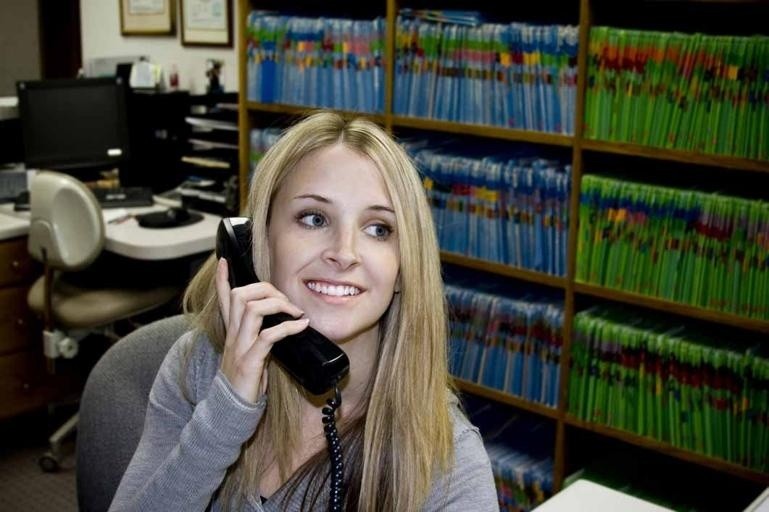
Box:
[[528, 478, 678, 512], [0, 182, 241, 420]]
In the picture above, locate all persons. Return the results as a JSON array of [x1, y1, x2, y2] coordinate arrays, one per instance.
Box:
[[109, 111, 501, 512]]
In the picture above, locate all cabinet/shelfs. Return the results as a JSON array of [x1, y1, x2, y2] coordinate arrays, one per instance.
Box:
[[235, 0, 769, 497]]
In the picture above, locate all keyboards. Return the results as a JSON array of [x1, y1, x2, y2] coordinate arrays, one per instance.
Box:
[[15, 187, 153, 212]]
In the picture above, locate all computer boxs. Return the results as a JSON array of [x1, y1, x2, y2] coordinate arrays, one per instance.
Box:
[[130, 90, 190, 191]]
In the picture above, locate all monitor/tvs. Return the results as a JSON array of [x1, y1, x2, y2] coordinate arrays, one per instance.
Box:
[[14, 77, 128, 183]]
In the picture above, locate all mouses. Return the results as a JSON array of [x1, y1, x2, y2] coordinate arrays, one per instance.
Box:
[[163, 206, 191, 226]]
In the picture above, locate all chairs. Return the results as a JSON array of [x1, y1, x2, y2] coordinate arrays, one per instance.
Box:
[[24, 170, 174, 475], [73, 313, 201, 512]]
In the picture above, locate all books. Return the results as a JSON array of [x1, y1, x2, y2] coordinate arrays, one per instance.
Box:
[[577, 174, 769, 321], [440, 285, 564, 407], [484, 438, 553, 510], [569, 306, 769, 474], [243, 9, 387, 175], [395, 10, 578, 134], [392, 139, 571, 276], [583, 27, 769, 163]]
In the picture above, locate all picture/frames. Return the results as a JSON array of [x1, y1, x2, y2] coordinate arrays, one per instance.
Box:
[[118, 0, 177, 37], [178, 0, 234, 50]]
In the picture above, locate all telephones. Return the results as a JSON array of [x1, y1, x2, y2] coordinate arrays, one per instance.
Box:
[[217, 216, 350, 395]]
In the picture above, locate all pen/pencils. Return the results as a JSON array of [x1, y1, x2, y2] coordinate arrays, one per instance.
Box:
[[109, 215, 133, 224]]
